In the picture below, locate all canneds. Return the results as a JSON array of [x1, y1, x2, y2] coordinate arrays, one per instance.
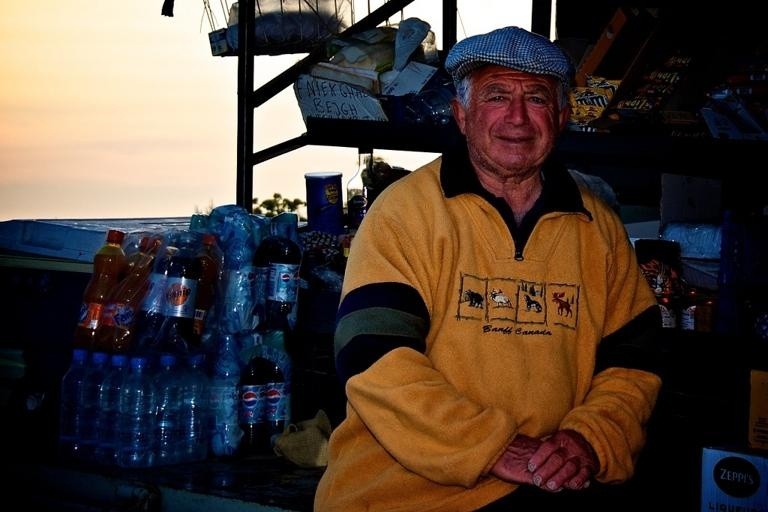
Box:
[[634, 238, 716, 332]]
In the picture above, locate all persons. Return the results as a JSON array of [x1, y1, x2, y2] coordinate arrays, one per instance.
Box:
[[314, 23, 664, 512]]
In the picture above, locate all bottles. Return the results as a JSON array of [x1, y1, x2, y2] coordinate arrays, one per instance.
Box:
[[63, 203, 303, 468]]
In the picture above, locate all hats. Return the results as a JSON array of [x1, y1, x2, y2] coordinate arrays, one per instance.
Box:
[[445, 26, 570, 93]]
